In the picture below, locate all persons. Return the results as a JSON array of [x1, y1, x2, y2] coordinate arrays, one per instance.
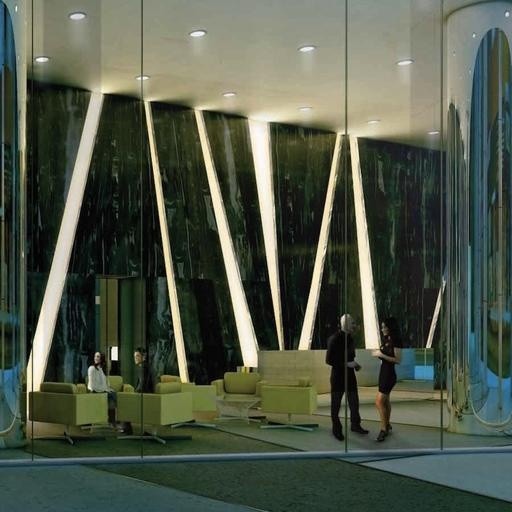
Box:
[[85, 351, 118, 425], [119, 347, 157, 432], [324, 313, 369, 440], [372, 317, 403, 443]]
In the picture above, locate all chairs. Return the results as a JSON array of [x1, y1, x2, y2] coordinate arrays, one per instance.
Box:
[[29, 373, 318, 444]]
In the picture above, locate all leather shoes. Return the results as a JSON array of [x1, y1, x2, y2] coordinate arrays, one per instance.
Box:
[[375, 424, 392, 441], [335, 432, 344, 441], [351, 427, 367, 434]]
